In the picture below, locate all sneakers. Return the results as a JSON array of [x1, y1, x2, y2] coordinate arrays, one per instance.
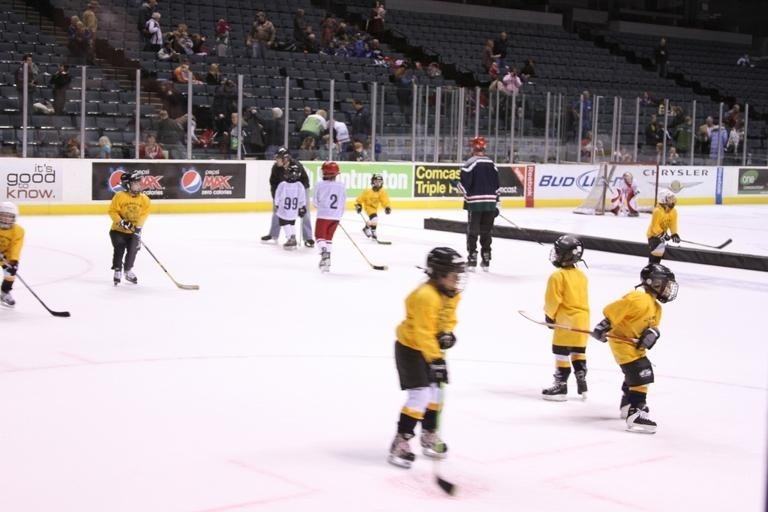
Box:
[[261, 234, 275, 240], [543, 374, 568, 395], [319, 253, 327, 267], [419, 427, 448, 453], [466, 251, 477, 266], [388, 424, 415, 461], [620, 396, 649, 417], [306, 239, 314, 246], [113, 267, 122, 282], [0, 289, 15, 305], [626, 408, 657, 429], [576, 372, 587, 393], [370, 225, 377, 237], [284, 235, 297, 246], [480, 249, 491, 267], [363, 225, 371, 238], [123, 269, 137, 281], [327, 251, 331, 265]]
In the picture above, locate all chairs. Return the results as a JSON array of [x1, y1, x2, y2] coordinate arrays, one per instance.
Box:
[[22, 32, 38, 43], [102, 131, 126, 147], [209, 85, 217, 92], [2, 99, 21, 113], [254, 78, 270, 89], [323, 91, 330, 101], [283, 67, 297, 77], [277, 51, 291, 57], [173, 82, 188, 95], [250, 66, 264, 76], [15, 41, 35, 52], [220, 63, 235, 73], [290, 99, 305, 111], [383, 7, 768, 145], [349, 72, 363, 81], [309, 62, 322, 70], [16, 51, 32, 60], [84, 132, 100, 149], [121, 104, 134, 117], [123, 132, 135, 143], [208, 96, 215, 106], [23, 24, 40, 33], [50, 54, 63, 64], [38, 63, 47, 73], [271, 88, 283, 97], [35, 42, 48, 53], [91, 0, 369, 49], [70, 79, 82, 90], [70, 67, 83, 78], [2, 86, 20, 99], [349, 82, 366, 93], [333, 72, 346, 81], [191, 54, 206, 62], [155, 60, 170, 69], [339, 92, 353, 103], [141, 105, 160, 117], [157, 72, 172, 80], [19, 115, 36, 129], [0, 50, 11, 60], [304, 80, 319, 90], [192, 96, 208, 107], [291, 90, 305, 99], [85, 103, 104, 115], [255, 88, 270, 96], [0, 114, 16, 129], [32, 115, 55, 129], [87, 79, 104, 91], [103, 104, 123, 116], [105, 92, 119, 104], [324, 63, 335, 70], [121, 93, 136, 105], [3, 32, 18, 43], [350, 64, 364, 72], [64, 100, 81, 115], [256, 97, 273, 109], [269, 78, 284, 89], [354, 93, 369, 103], [347, 56, 359, 63], [36, 52, 50, 62], [8, 23, 22, 32], [289, 79, 302, 90], [48, 64, 60, 75], [96, 116, 123, 131], [59, 130, 77, 145], [292, 52, 305, 59], [116, 117, 132, 130], [8, 64, 19, 72], [279, 57, 292, 65], [264, 50, 277, 56], [243, 87, 254, 97], [250, 56, 264, 66], [317, 70, 330, 79], [16, 129, 36, 156], [333, 56, 344, 62], [337, 63, 349, 71], [266, 56, 278, 67], [192, 84, 207, 93], [265, 66, 280, 75], [273, 99, 284, 107], [235, 65, 250, 73], [55, 115, 77, 130], [140, 51, 156, 60], [321, 54, 332, 61], [102, 80, 123, 92], [220, 55, 234, 62], [243, 98, 256, 107], [74, 115, 99, 131], [9, 12, 21, 24], [364, 67, 376, 74], [217, 91, 218, 91], [0, 129, 16, 146], [306, 53, 319, 60], [123, 50, 139, 60], [236, 55, 250, 65], [302, 70, 317, 80], [39, 130, 62, 157], [190, 63, 205, 70], [294, 61, 307, 67], [304, 91, 320, 101], [39, 34, 56, 44], [66, 90, 83, 101], [242, 74, 254, 86], [141, 61, 155, 71], [85, 91, 104, 103], [319, 81, 331, 90], [0, 40, 15, 51], [335, 82, 349, 91], [40, 88, 55, 102]]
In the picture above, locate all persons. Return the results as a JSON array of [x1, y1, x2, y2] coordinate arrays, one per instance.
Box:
[[592, 264, 678, 435], [158, 41, 181, 63], [226, 79, 235, 113], [296, 107, 311, 128], [137, 0, 157, 51], [520, 58, 534, 82], [71, 22, 92, 43], [147, 12, 162, 51], [138, 135, 164, 159], [213, 86, 227, 132], [228, 113, 246, 151], [482, 40, 501, 70], [573, 90, 592, 132], [332, 120, 349, 154], [17, 53, 39, 115], [63, 133, 89, 159], [165, 24, 194, 51], [216, 19, 228, 44], [294, 8, 307, 41], [388, 247, 466, 469], [207, 63, 220, 85], [215, 27, 232, 56], [252, 107, 285, 159], [246, 11, 276, 59], [243, 105, 266, 148], [0, 202, 25, 306], [313, 161, 347, 272], [67, 15, 82, 43], [454, 138, 500, 270], [639, 89, 745, 165], [304, 33, 322, 54], [494, 31, 509, 66], [274, 163, 306, 249], [181, 64, 201, 84], [261, 147, 315, 248], [321, 2, 443, 98], [611, 172, 640, 217], [351, 99, 372, 140], [737, 53, 755, 68], [352, 142, 366, 162], [646, 190, 680, 264], [355, 175, 392, 239], [190, 34, 213, 56], [652, 38, 669, 77], [503, 67, 520, 94], [109, 170, 151, 285], [490, 55, 501, 80], [83, 2, 99, 66], [159, 111, 183, 143], [540, 235, 591, 403], [301, 110, 328, 144], [176, 112, 201, 144], [95, 135, 113, 159], [175, 57, 193, 83], [48, 62, 71, 114]]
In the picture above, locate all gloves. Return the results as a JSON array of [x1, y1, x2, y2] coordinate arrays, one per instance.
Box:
[[7, 259, 18, 276], [430, 358, 450, 387], [298, 206, 307, 217], [671, 234, 680, 243], [133, 225, 140, 235], [593, 319, 611, 341], [659, 232, 668, 242], [120, 218, 131, 228], [636, 326, 660, 349], [436, 330, 455, 348], [545, 315, 555, 329], [386, 208, 390, 213], [0, 251, 8, 267], [354, 204, 363, 213]]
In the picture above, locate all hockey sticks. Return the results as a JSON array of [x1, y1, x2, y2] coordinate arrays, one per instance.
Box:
[[519, 310, 639, 345], [2, 258, 71, 317], [668, 235, 732, 250], [138, 236, 200, 291], [359, 211, 392, 246], [337, 222, 389, 272], [431, 349, 458, 499]]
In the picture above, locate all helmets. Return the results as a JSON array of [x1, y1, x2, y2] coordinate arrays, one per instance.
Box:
[[371, 174, 384, 191], [0, 202, 18, 229], [121, 170, 143, 194], [659, 191, 678, 209], [550, 234, 584, 266], [640, 263, 678, 303], [275, 146, 290, 167], [624, 171, 633, 187], [426, 246, 467, 297], [321, 161, 341, 178], [284, 163, 300, 182], [466, 136, 487, 154]]
